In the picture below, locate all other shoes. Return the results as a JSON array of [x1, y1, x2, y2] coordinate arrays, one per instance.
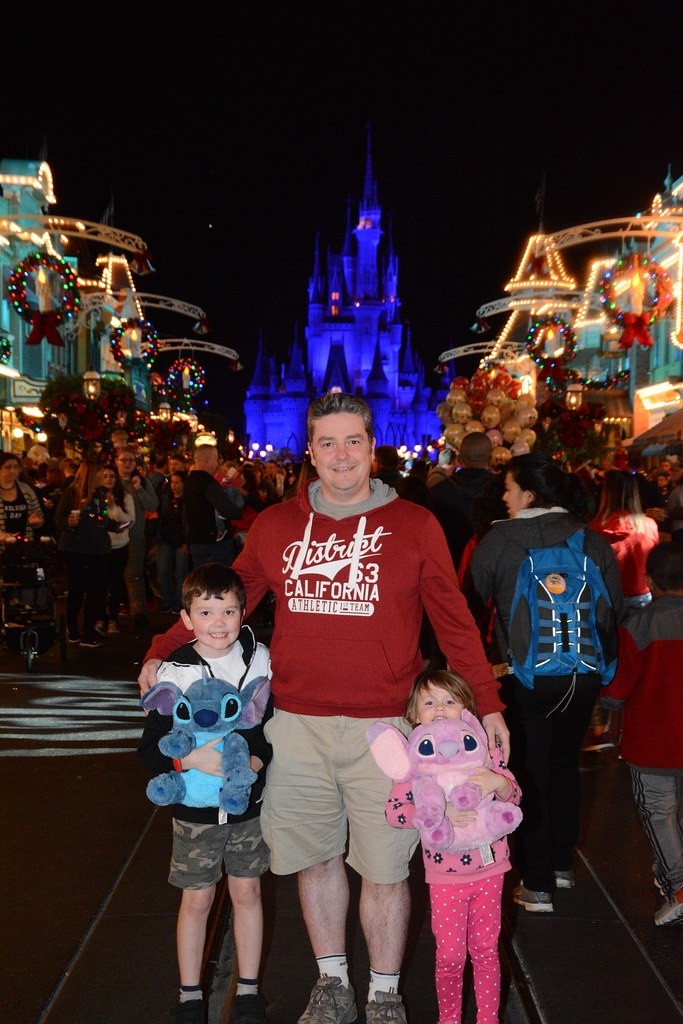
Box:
[[230, 993, 267, 1024], [106, 619, 120, 634], [80, 632, 104, 648], [655, 885, 683, 927], [553, 871, 585, 889], [67, 632, 82, 643], [512, 885, 555, 913], [94, 618, 105, 633], [134, 613, 152, 639], [176, 996, 208, 1024], [148, 576, 166, 600], [160, 604, 181, 615]]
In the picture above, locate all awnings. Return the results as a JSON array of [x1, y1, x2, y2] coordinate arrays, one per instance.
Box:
[[633, 407, 683, 448]]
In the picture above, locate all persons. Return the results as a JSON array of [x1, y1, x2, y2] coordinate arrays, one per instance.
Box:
[[384, 671, 523, 1024], [595, 538, 683, 928], [0, 427, 683, 648], [463, 456, 625, 913], [573, 466, 663, 753], [139, 565, 275, 1024], [138, 393, 511, 1024]]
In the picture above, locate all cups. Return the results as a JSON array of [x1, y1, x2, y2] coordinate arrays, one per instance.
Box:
[[71, 510, 80, 523]]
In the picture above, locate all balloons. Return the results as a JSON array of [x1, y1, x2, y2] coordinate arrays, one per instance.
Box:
[[435, 367, 540, 469]]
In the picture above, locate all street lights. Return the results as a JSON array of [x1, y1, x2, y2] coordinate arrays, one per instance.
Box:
[[82, 370, 102, 504]]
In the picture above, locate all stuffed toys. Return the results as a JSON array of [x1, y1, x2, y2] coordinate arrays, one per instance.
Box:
[[365, 709, 523, 866], [139, 675, 269, 825]]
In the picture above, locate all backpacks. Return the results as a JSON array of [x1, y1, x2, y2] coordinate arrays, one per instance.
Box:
[[497, 527, 619, 692]]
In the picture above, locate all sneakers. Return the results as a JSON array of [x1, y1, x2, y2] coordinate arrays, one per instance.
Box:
[[365, 990, 407, 1024], [297, 977, 358, 1024]]
